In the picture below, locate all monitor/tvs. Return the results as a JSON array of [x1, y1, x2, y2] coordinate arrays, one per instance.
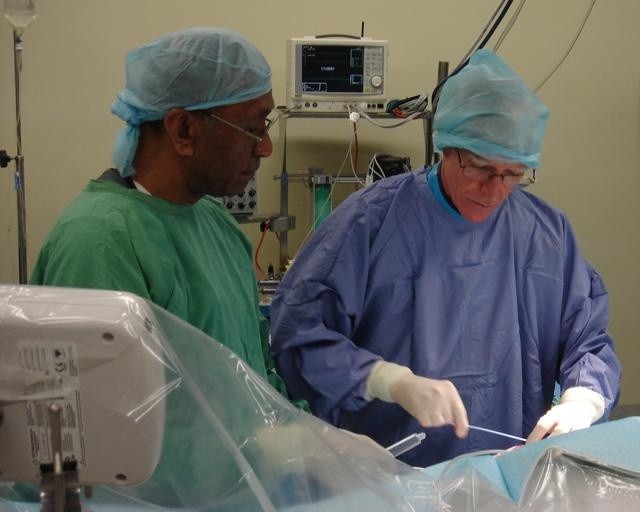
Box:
[[288, 39, 388, 112]]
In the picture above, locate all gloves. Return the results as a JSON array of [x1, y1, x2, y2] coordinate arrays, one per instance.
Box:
[[369, 360, 470, 441], [527, 387, 607, 444]]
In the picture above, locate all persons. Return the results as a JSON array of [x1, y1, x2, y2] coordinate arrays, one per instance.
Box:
[[268, 48, 626, 470], [25, 26, 327, 512]]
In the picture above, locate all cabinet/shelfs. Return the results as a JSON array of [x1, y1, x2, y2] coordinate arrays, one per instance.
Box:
[[276, 104, 434, 280]]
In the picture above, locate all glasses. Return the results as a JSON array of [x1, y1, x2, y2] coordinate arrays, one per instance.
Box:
[[205, 110, 271, 144], [454, 150, 538, 191]]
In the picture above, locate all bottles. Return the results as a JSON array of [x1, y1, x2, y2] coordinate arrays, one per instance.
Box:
[[4, 1, 37, 34]]
